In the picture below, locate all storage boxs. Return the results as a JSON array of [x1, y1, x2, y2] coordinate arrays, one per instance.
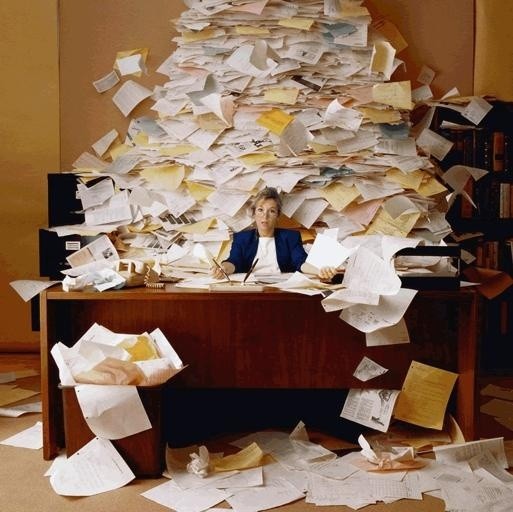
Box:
[[59, 382, 167, 480]]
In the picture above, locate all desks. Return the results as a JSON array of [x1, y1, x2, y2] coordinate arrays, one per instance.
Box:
[[39, 285, 482, 460]]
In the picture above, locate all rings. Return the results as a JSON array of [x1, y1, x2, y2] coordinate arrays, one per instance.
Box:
[[328, 271, 333, 276]]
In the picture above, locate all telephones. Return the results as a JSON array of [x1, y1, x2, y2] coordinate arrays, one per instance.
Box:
[[115, 259, 166, 288]]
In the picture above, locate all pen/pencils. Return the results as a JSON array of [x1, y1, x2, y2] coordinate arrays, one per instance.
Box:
[[244, 258, 259, 283], [212, 259, 231, 281]]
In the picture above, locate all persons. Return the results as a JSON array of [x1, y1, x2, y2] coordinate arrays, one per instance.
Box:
[[207, 186, 339, 280]]
